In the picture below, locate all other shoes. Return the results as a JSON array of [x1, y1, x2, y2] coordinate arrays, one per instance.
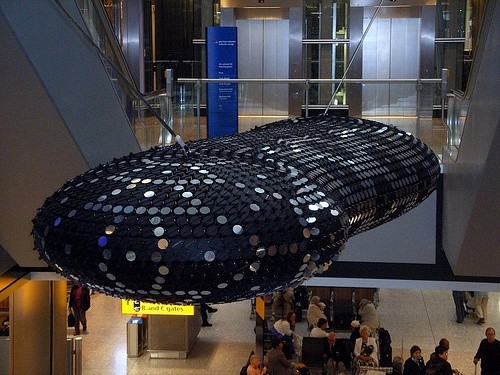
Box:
[[208, 308, 218, 313], [456, 320, 462, 323], [73, 332, 80, 335], [202, 323, 212, 327], [475, 318, 486, 324], [83, 331, 89, 334]]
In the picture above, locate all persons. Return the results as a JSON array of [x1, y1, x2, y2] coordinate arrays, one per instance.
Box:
[[68, 284, 90, 335], [247, 355, 266, 375], [271, 288, 293, 320], [309, 318, 329, 338], [306, 296, 329, 329], [2, 316, 9, 336], [452, 290, 489, 325], [386, 356, 403, 375], [322, 333, 348, 375], [198, 303, 218, 327], [354, 326, 379, 367], [269, 312, 296, 360], [474, 328, 500, 375], [403, 338, 457, 375], [358, 299, 378, 330], [267, 338, 310, 375], [350, 320, 371, 358]]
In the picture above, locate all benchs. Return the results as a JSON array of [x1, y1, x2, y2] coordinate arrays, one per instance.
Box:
[[250, 298, 297, 321]]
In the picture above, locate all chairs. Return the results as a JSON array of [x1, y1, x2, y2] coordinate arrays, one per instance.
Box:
[[300, 335, 331, 369], [331, 338, 353, 370]]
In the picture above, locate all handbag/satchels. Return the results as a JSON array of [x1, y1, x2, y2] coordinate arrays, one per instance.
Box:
[[362, 343, 374, 356], [68, 313, 76, 327]]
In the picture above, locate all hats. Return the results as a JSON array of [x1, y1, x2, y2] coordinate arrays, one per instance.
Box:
[[350, 320, 360, 328]]
